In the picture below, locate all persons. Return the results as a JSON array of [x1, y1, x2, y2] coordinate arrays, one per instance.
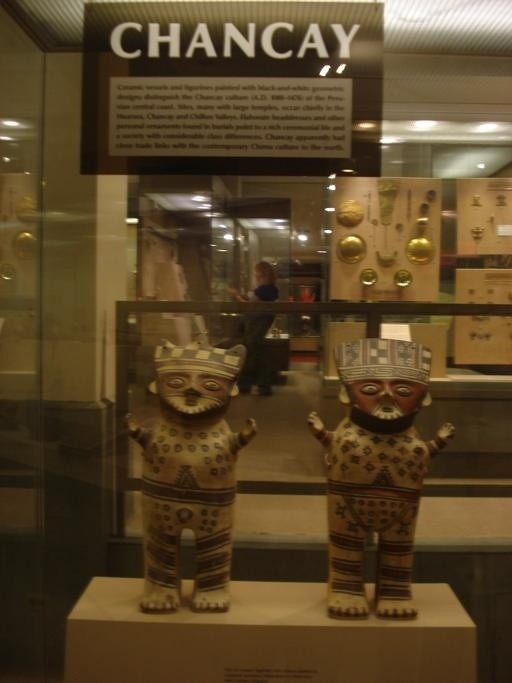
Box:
[[223, 258, 282, 396]]
